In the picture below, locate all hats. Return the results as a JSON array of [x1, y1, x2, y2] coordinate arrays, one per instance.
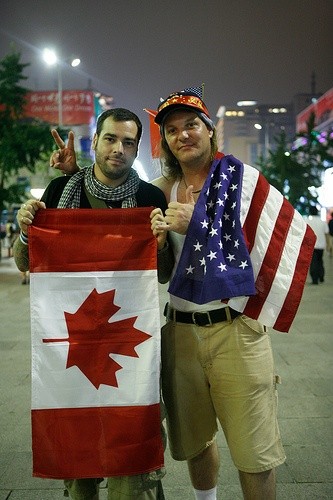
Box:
[[155, 92, 211, 123]]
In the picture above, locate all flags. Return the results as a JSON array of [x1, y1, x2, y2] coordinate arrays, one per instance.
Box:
[[168, 150, 318, 333], [176, 83, 205, 100], [144, 109, 176, 159], [28, 206, 165, 481]]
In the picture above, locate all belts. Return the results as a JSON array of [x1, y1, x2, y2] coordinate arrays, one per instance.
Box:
[[164, 302, 241, 327]]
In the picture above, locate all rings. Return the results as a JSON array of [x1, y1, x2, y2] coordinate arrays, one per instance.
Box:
[[21, 216, 24, 222]]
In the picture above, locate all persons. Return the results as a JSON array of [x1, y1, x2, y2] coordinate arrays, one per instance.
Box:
[[302, 206, 329, 285], [50, 92, 287, 500], [327, 212, 333, 258], [14, 108, 173, 500]]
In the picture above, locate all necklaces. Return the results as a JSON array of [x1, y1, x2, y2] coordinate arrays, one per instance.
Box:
[[183, 176, 206, 194]]
